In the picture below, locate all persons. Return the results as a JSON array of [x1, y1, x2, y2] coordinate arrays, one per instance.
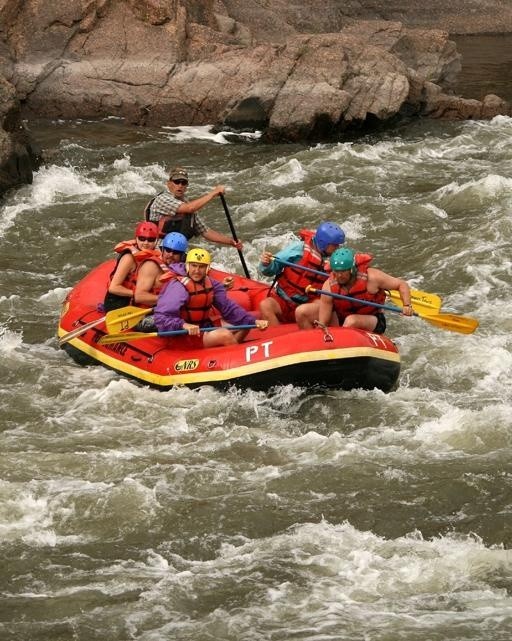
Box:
[[259, 222, 345, 326], [144, 169, 243, 254], [295, 247, 414, 335], [104, 222, 160, 312], [153, 248, 268, 349], [131, 232, 234, 333]]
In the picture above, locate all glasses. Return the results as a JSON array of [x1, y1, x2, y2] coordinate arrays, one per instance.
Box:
[[137, 235, 157, 242], [170, 179, 188, 186]]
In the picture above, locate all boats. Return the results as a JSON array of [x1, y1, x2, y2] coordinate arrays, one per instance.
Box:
[[59, 257, 402, 394]]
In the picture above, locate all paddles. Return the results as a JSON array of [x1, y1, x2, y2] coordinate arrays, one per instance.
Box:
[[268, 255, 441, 316], [97, 324, 259, 346], [57, 316, 105, 348], [303, 285, 477, 335], [105, 276, 233, 332]]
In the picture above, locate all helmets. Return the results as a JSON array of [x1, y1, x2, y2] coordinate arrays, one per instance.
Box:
[[134, 221, 159, 239], [185, 248, 211, 277], [161, 231, 188, 254], [315, 221, 345, 253], [329, 248, 353, 270]]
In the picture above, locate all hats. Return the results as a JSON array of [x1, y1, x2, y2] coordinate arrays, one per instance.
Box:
[[169, 168, 189, 184]]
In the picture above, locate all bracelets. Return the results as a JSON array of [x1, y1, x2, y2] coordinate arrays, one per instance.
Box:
[[403, 304, 412, 308]]
